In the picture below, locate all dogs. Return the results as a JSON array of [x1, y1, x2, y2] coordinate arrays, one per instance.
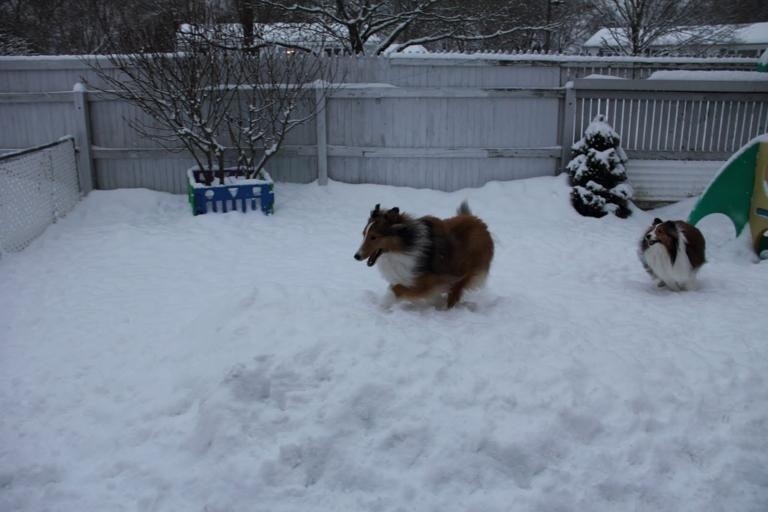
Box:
[[637, 217, 705, 291], [354, 198, 497, 311]]
